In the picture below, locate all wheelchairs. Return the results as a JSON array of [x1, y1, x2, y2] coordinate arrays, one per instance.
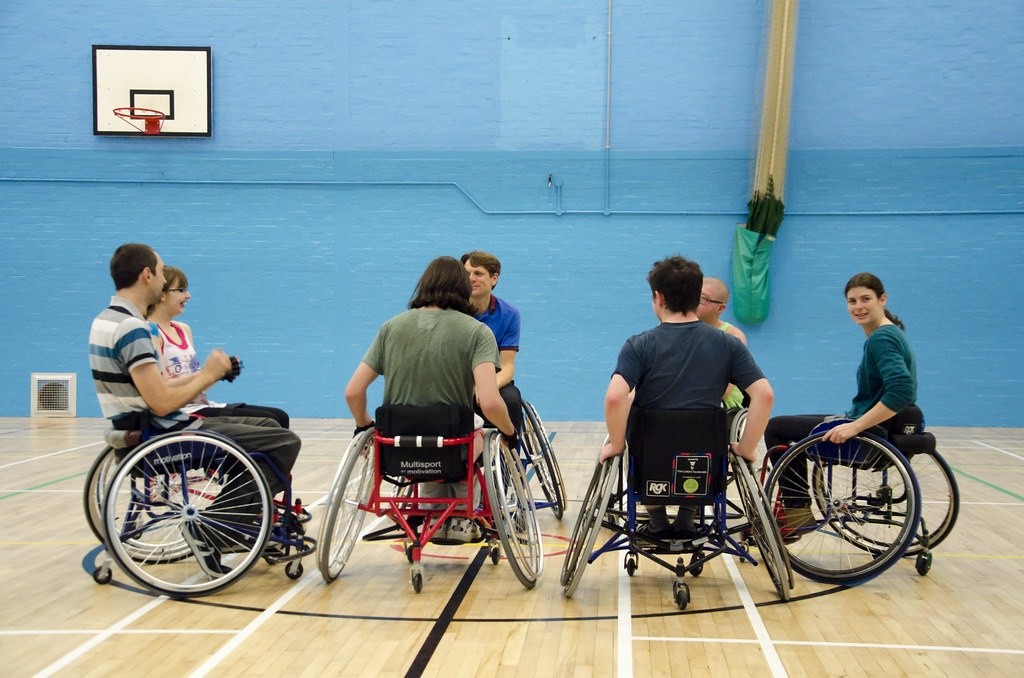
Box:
[[738, 403, 960, 586], [314, 399, 568, 594], [82, 427, 319, 586], [557, 403, 795, 612]]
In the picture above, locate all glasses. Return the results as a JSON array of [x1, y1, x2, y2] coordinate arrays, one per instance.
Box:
[[167, 288, 186, 292], [700, 296, 723, 305]]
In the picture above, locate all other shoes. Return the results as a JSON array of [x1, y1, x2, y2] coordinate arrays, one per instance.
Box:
[[671, 522, 709, 538], [638, 523, 672, 539], [447, 518, 481, 542], [423, 518, 445, 538], [779, 507, 816, 537]]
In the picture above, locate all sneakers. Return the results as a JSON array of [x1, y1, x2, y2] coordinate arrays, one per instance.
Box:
[[178, 520, 233, 578], [221, 532, 278, 554]]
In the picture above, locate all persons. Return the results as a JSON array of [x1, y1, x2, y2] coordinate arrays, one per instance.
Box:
[[89, 243, 300, 577], [600, 256, 773, 544], [765, 273, 917, 535], [344, 252, 524, 541]]
